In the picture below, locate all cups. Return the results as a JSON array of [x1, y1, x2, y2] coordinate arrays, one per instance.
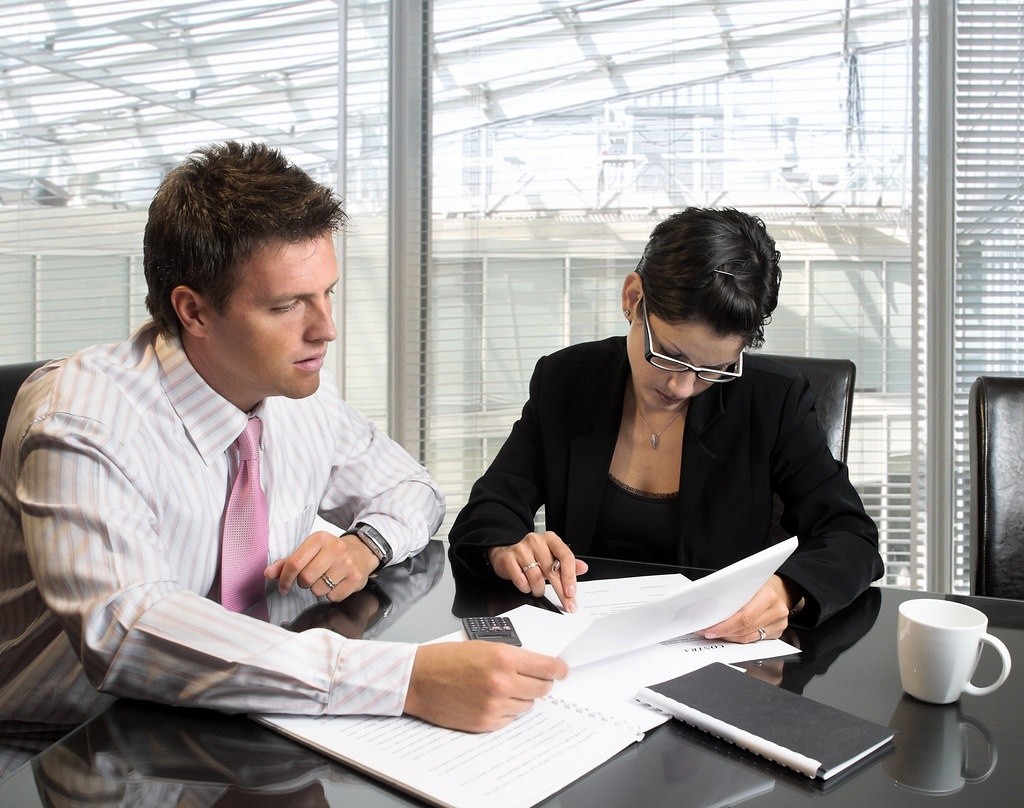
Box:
[[896, 597, 1011, 703], [879, 692, 999, 796]]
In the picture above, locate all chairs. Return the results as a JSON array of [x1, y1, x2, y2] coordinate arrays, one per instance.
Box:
[[968, 376, 1024, 599], [745, 353, 856, 464]]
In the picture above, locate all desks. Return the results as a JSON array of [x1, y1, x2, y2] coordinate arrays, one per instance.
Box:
[[0, 549, 1024, 808]]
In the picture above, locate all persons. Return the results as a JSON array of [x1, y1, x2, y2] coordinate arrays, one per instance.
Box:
[[446, 208, 886, 644], [0, 141, 568, 732]]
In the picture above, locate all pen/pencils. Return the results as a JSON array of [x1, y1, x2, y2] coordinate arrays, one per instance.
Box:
[[552, 559, 560, 572]]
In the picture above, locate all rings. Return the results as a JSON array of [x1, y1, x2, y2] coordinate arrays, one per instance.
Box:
[[758, 628, 767, 640], [322, 572, 336, 588], [523, 562, 538, 571]]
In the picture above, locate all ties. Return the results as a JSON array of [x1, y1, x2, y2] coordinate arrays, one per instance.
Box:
[[221, 417, 270, 624]]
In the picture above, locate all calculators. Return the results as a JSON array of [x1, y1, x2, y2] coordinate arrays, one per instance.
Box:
[[461, 617, 522, 647]]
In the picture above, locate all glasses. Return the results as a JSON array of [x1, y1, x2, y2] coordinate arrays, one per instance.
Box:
[[637, 268, 743, 382]]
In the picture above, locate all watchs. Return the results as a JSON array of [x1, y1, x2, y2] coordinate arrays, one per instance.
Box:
[[340, 524, 393, 576]]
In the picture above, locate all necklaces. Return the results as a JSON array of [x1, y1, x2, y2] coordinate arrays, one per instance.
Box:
[[628, 380, 689, 450]]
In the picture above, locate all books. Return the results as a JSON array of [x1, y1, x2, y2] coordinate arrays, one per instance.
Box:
[[253, 601, 746, 808], [635, 662, 896, 780]]
